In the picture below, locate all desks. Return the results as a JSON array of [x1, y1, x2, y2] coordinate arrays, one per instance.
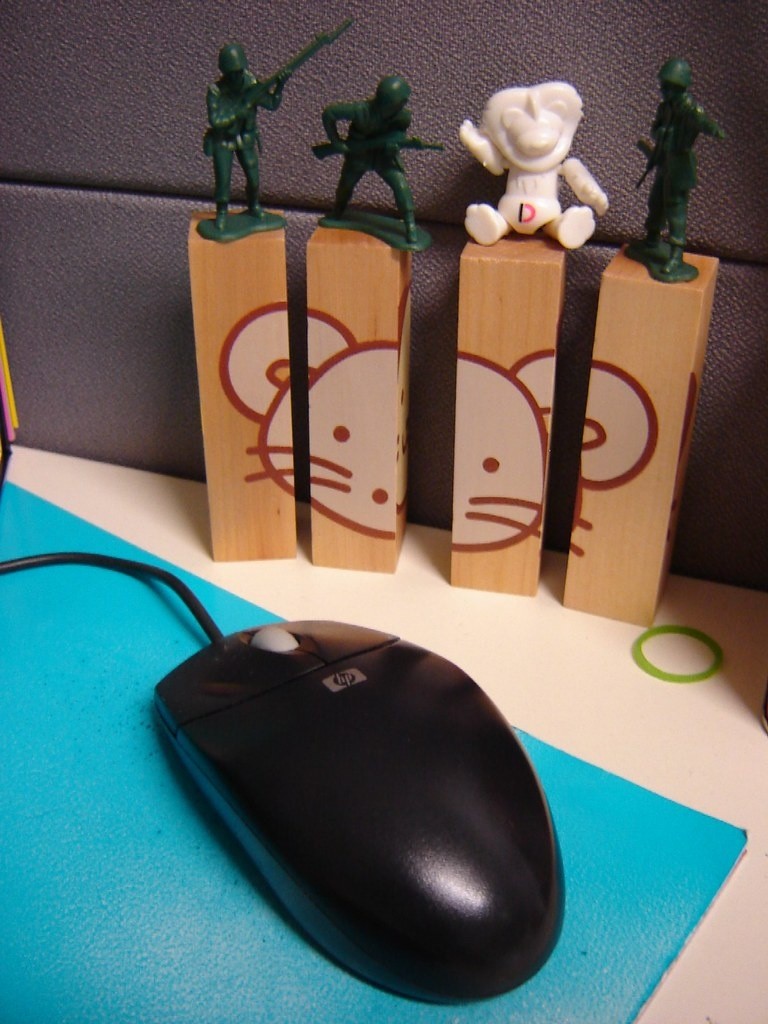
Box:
[[0, 444, 767, 1024]]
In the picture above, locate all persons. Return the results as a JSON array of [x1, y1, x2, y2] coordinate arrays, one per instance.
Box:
[[204, 16, 292, 229], [312, 78, 447, 244], [633, 56, 727, 276]]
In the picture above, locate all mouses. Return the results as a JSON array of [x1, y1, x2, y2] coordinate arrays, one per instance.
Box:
[[151, 620, 567, 1005]]
